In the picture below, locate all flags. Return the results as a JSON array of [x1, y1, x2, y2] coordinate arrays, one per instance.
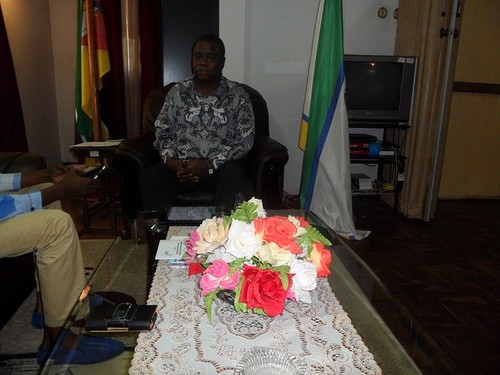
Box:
[[298, 0, 373, 241], [74, 0, 111, 167]]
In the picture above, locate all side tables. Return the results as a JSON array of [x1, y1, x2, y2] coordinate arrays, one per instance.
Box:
[[69, 140, 123, 232]]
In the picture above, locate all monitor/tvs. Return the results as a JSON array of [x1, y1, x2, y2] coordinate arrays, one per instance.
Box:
[[343, 54, 418, 123]]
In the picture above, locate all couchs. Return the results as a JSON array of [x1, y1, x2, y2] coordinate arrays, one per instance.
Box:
[[113, 81, 289, 230], [0, 153, 50, 331]]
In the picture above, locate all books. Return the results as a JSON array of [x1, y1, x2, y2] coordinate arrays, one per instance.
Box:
[[154, 235, 189, 267]]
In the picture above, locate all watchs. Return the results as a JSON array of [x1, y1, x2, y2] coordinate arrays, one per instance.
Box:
[[201, 158, 214, 176]]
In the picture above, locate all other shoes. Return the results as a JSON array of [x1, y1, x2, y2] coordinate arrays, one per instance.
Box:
[[37, 334, 125, 366], [31, 293, 103, 328]]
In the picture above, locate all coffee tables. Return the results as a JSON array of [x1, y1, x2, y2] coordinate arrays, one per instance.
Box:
[[40, 207, 464, 375]]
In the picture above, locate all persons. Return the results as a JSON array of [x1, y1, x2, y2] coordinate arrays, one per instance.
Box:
[[1, 164, 126, 367], [138, 33, 255, 273]]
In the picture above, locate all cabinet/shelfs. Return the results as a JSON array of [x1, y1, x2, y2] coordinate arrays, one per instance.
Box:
[[348, 123, 410, 210]]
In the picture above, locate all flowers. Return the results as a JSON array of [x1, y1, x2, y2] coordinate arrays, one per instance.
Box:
[[183, 197, 331, 323]]
